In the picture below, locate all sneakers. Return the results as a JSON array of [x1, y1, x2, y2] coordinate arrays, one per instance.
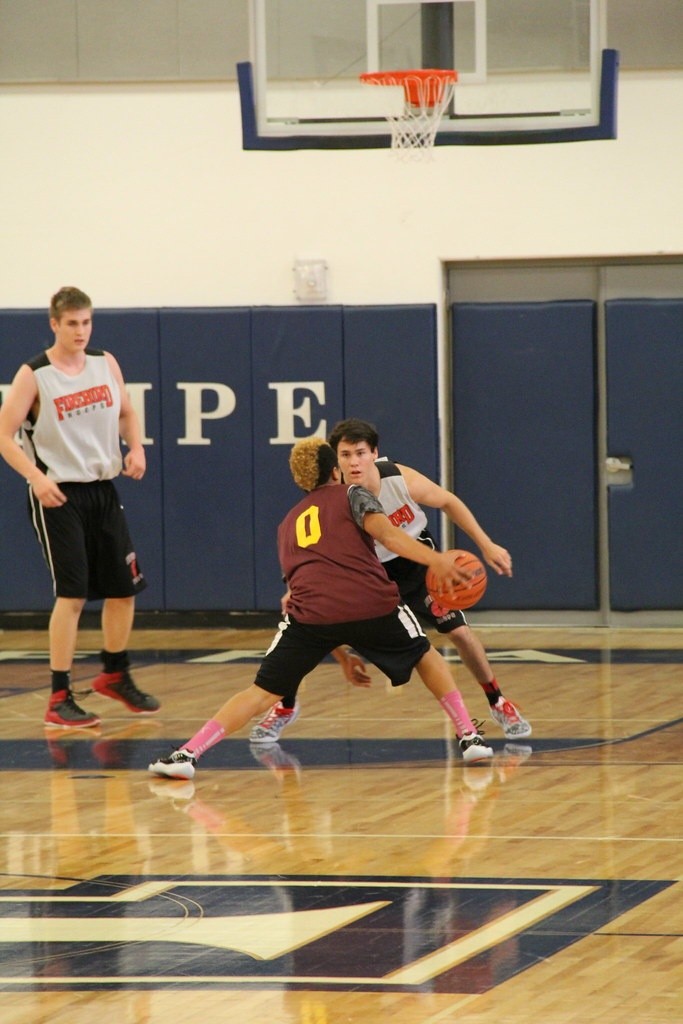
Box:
[[455, 718, 494, 764], [148, 750, 198, 780], [40, 727, 101, 764], [249, 743, 302, 787], [89, 669, 162, 714], [498, 743, 532, 783], [134, 775, 195, 802], [91, 718, 166, 763], [489, 696, 531, 739], [460, 763, 493, 799], [249, 698, 300, 742], [43, 693, 100, 729]]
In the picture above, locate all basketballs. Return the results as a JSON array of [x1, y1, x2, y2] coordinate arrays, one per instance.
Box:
[[425, 549, 487, 611]]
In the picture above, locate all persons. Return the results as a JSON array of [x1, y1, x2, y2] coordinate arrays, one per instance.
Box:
[[248, 417, 533, 743], [0, 286, 162, 725], [148, 438, 496, 778]]
